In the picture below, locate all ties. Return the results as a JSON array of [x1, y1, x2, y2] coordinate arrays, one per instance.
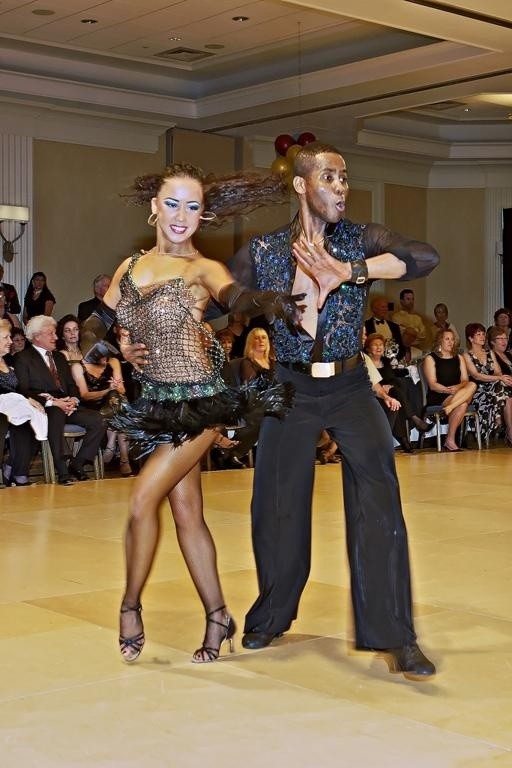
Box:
[[47, 351, 63, 389]]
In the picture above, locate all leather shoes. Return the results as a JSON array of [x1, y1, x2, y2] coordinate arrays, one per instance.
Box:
[[70, 464, 89, 482], [227, 453, 247, 469], [242, 624, 287, 649], [383, 640, 437, 682]]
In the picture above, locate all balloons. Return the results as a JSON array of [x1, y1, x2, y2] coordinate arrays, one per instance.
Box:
[[297, 132, 316, 146], [272, 157, 288, 175], [286, 144, 303, 165], [275, 134, 296, 156]]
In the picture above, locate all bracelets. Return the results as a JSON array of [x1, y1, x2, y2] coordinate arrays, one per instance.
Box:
[[349, 259, 369, 285]]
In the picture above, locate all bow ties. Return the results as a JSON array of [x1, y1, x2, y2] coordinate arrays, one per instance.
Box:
[[375, 319, 385, 325]]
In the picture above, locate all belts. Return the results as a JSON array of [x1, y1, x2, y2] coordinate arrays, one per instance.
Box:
[[277, 356, 364, 379]]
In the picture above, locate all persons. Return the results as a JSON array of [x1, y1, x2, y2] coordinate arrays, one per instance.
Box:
[[241, 326, 272, 390], [23, 271, 56, 326], [0, 317, 44, 487], [364, 332, 434, 453], [423, 329, 477, 452], [494, 308, 512, 352], [13, 315, 105, 486], [57, 314, 85, 365], [402, 327, 422, 362], [78, 274, 134, 403], [461, 323, 512, 448], [0, 265, 22, 315], [119, 141, 440, 680], [228, 309, 249, 358], [430, 303, 460, 352], [216, 329, 234, 362], [4, 327, 26, 366], [200, 322, 260, 470], [392, 289, 426, 349], [362, 296, 405, 361], [319, 440, 340, 463], [71, 356, 133, 477], [489, 327, 512, 375], [0, 289, 21, 327], [78, 162, 307, 667], [361, 326, 402, 412]]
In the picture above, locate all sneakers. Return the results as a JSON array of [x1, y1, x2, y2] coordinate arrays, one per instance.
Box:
[[12, 473, 34, 487], [2, 465, 13, 486]]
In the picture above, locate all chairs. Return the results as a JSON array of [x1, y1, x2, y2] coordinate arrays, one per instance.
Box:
[[5, 430, 50, 484], [206, 425, 253, 471], [230, 358, 245, 386], [100, 439, 142, 479], [418, 362, 482, 451], [47, 423, 98, 483]]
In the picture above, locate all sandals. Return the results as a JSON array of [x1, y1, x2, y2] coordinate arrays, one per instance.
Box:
[[103, 445, 117, 468], [120, 459, 134, 478]]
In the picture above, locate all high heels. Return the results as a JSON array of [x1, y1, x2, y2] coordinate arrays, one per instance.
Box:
[[403, 444, 418, 452], [417, 421, 436, 437], [120, 598, 146, 661], [443, 445, 464, 452], [192, 604, 238, 663], [440, 407, 448, 422]]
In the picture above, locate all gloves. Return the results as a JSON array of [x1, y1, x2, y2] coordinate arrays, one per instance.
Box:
[[258, 289, 308, 326]]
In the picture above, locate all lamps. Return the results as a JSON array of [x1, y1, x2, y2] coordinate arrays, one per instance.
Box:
[[0, 205, 29, 262]]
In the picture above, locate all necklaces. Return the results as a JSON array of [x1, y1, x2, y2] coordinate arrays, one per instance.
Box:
[[33, 289, 42, 295], [299, 222, 325, 246], [139, 248, 199, 257]]
